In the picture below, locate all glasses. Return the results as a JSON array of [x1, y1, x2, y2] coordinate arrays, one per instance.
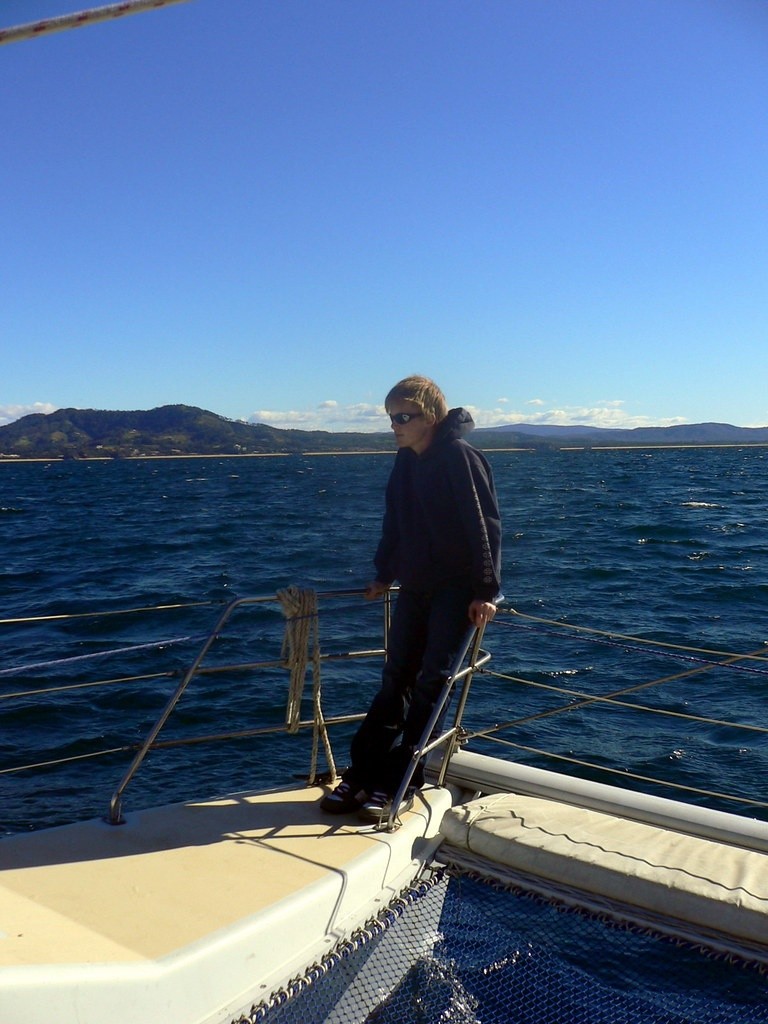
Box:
[[389, 413, 422, 424]]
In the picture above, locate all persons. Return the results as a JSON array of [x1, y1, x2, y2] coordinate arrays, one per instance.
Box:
[[320, 376, 503, 822]]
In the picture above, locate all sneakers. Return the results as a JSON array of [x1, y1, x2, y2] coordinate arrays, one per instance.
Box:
[[320, 778, 376, 814], [358, 786, 414, 823]]
